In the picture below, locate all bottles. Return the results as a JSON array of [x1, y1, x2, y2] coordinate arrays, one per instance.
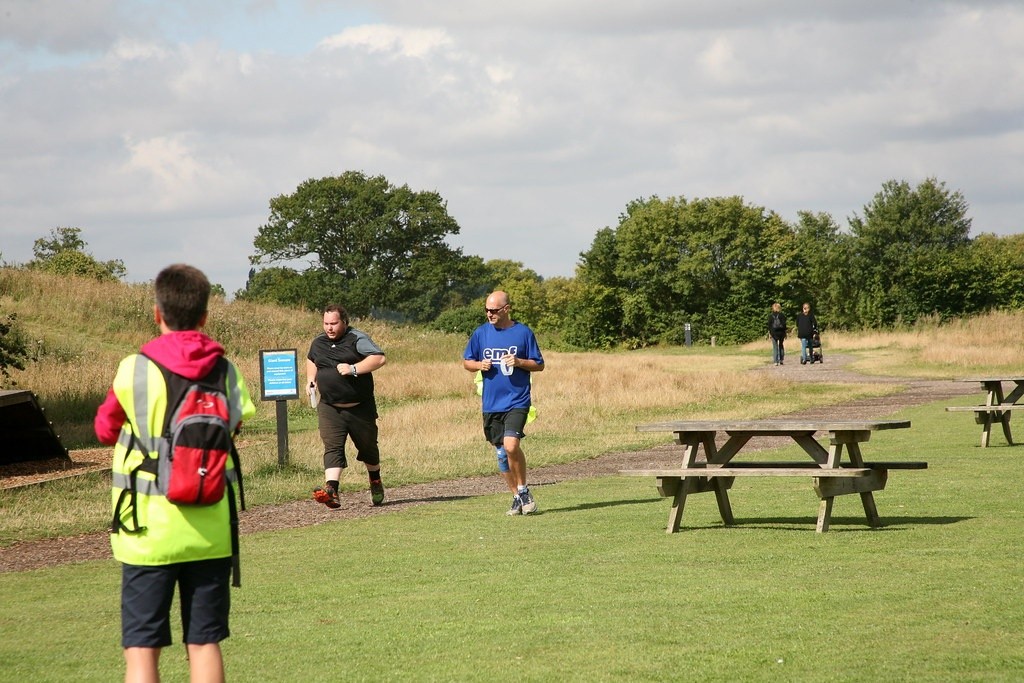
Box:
[[310, 381, 317, 409]]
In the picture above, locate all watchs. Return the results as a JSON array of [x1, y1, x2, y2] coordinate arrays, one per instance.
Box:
[[350, 365, 358, 377]]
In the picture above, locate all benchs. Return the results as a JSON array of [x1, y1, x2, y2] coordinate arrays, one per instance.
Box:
[[619, 468, 873, 534], [682, 460, 928, 528], [945, 405, 1024, 447]]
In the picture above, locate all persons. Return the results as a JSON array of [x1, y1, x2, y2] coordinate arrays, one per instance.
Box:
[[305, 304, 386, 509], [93, 262, 259, 683], [796, 302, 818, 364], [768, 303, 788, 366], [462, 290, 545, 517]]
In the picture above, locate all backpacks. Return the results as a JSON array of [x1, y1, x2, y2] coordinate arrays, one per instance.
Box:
[[138, 352, 230, 507], [770, 313, 785, 340]]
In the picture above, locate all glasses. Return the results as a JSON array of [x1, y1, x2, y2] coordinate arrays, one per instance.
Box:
[[484, 302, 509, 314]]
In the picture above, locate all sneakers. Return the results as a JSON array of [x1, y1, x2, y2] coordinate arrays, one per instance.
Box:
[[369, 474, 385, 505], [312, 485, 341, 509], [505, 496, 522, 516], [518, 485, 537, 514]]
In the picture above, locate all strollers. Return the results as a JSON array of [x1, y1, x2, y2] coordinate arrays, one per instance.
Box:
[[800, 328, 823, 364]]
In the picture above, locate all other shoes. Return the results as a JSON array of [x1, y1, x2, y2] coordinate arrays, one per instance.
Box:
[[775, 360, 783, 366], [802, 359, 814, 364]]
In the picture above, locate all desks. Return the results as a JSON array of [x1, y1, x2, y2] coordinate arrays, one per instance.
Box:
[[635, 420, 911, 535], [952, 376, 1024, 447]]
